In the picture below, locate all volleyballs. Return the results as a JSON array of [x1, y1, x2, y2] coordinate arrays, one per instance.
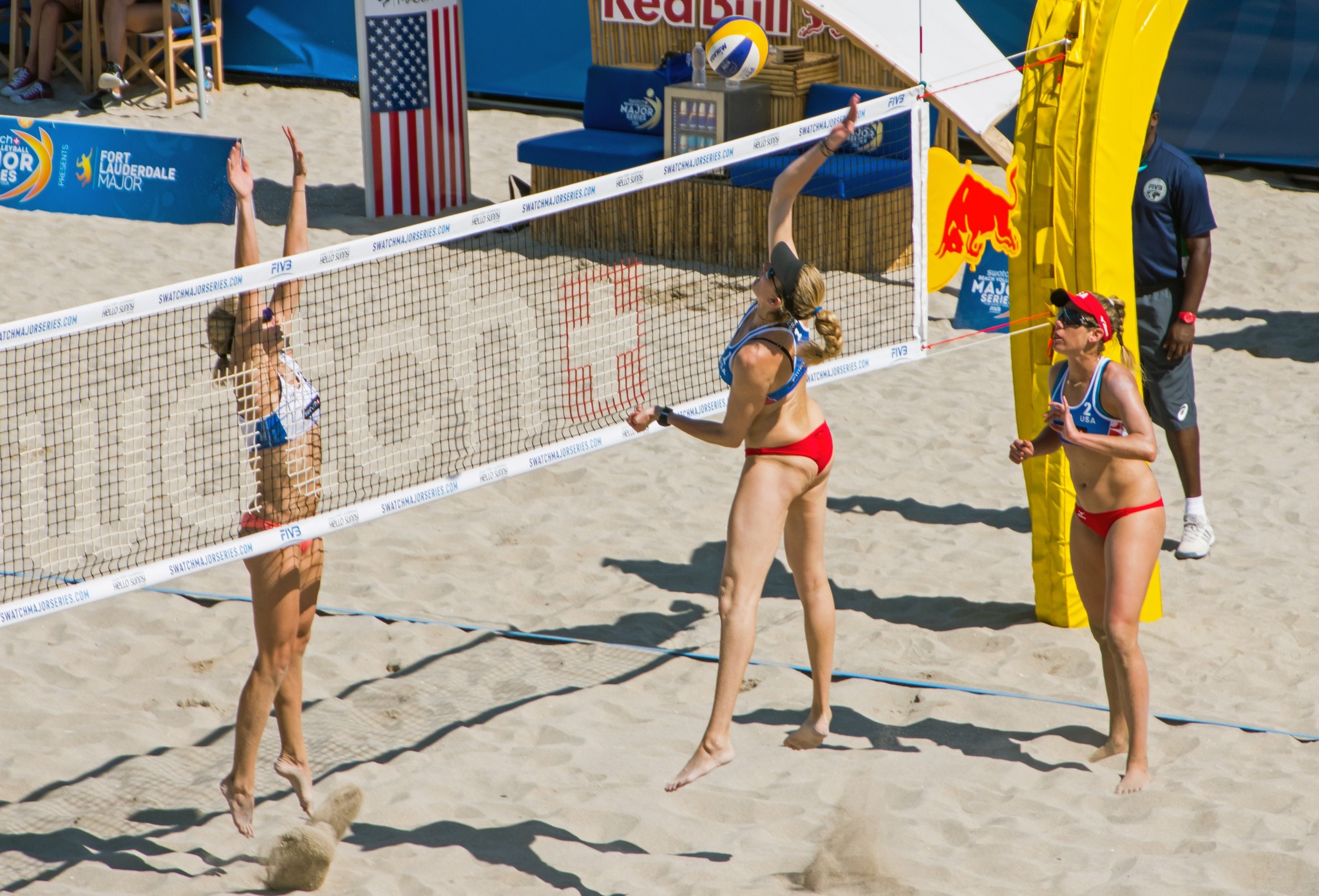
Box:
[[705, 14, 769, 81]]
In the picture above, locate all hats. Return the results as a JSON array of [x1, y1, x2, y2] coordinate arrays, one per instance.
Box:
[[1050, 287, 1112, 342], [771, 241, 807, 312]]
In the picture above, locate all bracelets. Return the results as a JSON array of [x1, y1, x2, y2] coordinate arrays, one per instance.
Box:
[[818, 136, 836, 158], [292, 190, 306, 192]]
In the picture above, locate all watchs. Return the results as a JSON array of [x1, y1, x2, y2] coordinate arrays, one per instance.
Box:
[[657, 406, 673, 427], [1178, 311, 1197, 325]]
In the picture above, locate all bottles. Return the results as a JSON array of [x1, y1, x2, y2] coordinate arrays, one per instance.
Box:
[[691, 41, 706, 85], [725, 79, 740, 88], [203, 66, 214, 105]]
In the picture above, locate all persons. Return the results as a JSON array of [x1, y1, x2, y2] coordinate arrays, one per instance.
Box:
[[78, 0, 191, 110], [0, 0, 82, 105], [627, 93, 859, 793], [204, 125, 326, 840], [1009, 288, 1168, 792], [1131, 90, 1218, 560]]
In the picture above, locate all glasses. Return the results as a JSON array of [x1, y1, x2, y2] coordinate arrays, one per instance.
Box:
[[1056, 307, 1099, 327], [766, 266, 782, 299], [262, 307, 274, 323]]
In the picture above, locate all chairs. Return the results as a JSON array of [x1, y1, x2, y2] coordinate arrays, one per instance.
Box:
[[0, 0, 91, 95], [513, 60, 944, 270], [88, 0, 224, 108]]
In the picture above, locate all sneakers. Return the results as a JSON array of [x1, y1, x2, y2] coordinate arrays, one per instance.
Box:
[[10, 80, 54, 104], [1, 67, 36, 96], [1175, 514, 1215, 560], [80, 91, 121, 110], [98, 61, 130, 89]]
